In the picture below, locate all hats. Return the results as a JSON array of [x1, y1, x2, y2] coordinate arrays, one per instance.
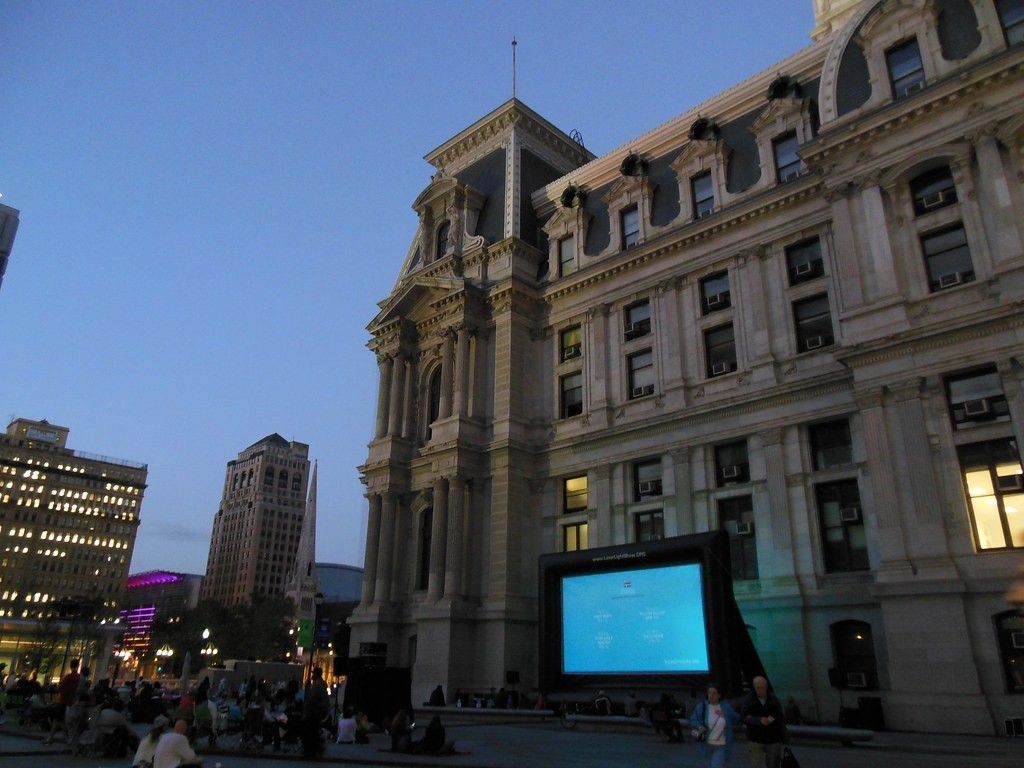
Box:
[[0, 663, 7, 667], [154, 715, 170, 729], [489, 687, 496, 691], [311, 667, 322, 675]]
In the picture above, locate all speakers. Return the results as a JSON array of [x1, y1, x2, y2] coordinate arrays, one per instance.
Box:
[[828, 668, 845, 686], [859, 697, 887, 731], [333, 657, 347, 676], [506, 671, 520, 684]]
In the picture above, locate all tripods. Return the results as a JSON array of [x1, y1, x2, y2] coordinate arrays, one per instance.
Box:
[[330, 676, 341, 724]]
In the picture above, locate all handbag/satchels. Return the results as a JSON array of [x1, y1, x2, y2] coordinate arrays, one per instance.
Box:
[[781, 747, 801, 768], [690, 701, 707, 742]]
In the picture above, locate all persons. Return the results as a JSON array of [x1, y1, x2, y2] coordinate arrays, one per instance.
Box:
[[0, 659, 456, 758], [450, 686, 698, 744], [418, 222, 431, 263], [132, 715, 205, 768], [785, 694, 803, 726], [446, 202, 460, 246], [690, 676, 788, 768]]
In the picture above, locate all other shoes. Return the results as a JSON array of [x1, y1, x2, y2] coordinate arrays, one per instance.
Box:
[[40, 740, 52, 746]]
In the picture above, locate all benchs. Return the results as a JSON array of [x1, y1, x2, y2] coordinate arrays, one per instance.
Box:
[[568, 714, 876, 749], [413, 706, 555, 723]]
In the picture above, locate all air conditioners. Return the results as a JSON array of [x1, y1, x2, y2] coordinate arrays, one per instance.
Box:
[[846, 672, 867, 687], [713, 362, 730, 376], [723, 465, 742, 479], [624, 322, 639, 336], [565, 346, 579, 357], [964, 399, 990, 416], [924, 191, 946, 209], [940, 272, 961, 288], [796, 260, 813, 277], [840, 508, 859, 521], [1012, 632, 1024, 649], [736, 521, 753, 535], [639, 482, 655, 494], [707, 293, 725, 307], [784, 170, 800, 183], [807, 335, 826, 349], [904, 81, 924, 96], [633, 385, 648, 397], [700, 208, 713, 218]]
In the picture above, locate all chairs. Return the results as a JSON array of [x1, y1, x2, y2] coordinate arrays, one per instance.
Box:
[[61, 702, 113, 760], [221, 720, 303, 754]]
